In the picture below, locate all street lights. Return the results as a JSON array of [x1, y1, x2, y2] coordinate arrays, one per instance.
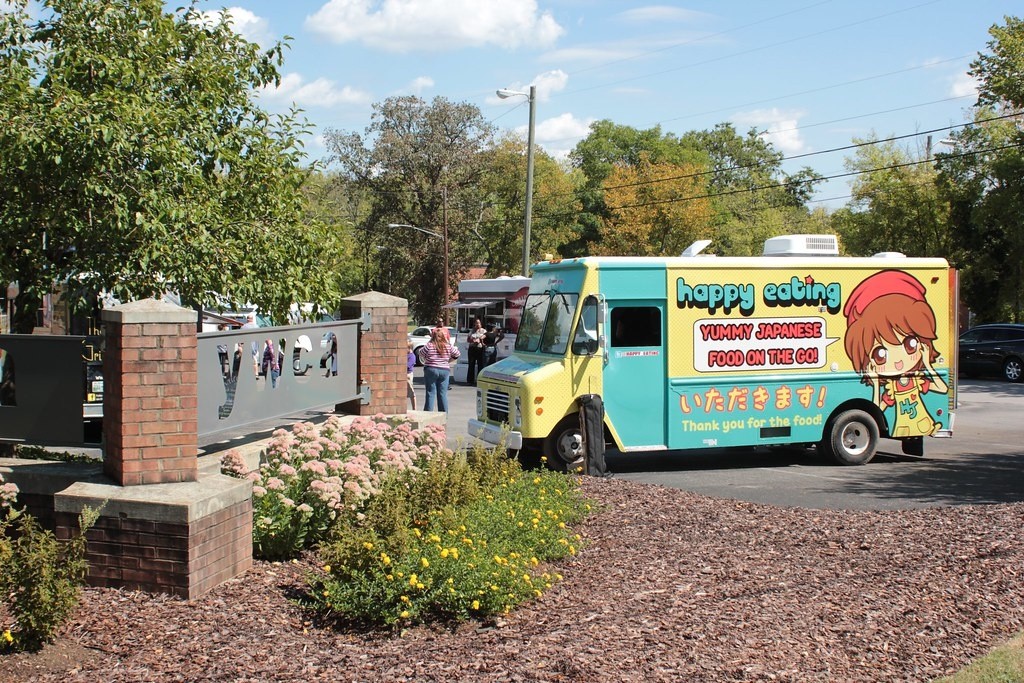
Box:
[[926, 134, 957, 172], [388, 187, 451, 327], [496, 85, 538, 277]]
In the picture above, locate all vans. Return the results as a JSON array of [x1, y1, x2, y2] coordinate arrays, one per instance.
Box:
[[466, 233, 961, 476]]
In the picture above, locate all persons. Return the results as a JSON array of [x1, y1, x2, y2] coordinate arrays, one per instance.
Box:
[[480, 322, 505, 368], [237, 314, 261, 380], [263, 335, 279, 389], [418, 330, 461, 412], [466, 319, 488, 386], [430, 318, 452, 391], [215, 324, 229, 377], [400, 339, 417, 410], [325, 332, 338, 378]]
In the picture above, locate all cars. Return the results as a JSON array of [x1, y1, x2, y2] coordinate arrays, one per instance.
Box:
[[406, 326, 456, 366], [956, 324, 1024, 383], [73, 268, 340, 335]]
